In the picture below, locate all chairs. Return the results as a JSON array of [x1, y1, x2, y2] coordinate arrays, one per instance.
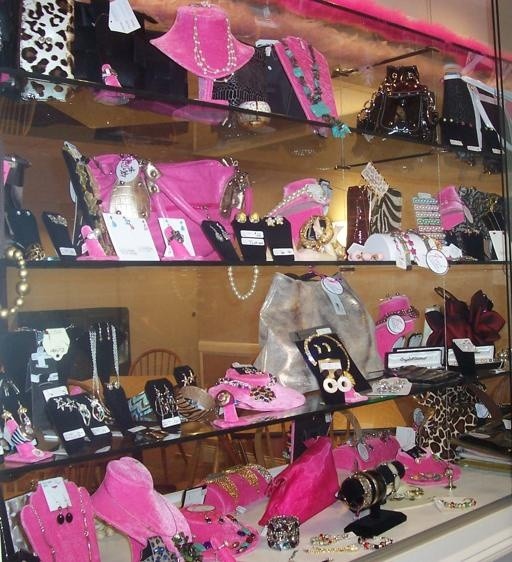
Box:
[[29, 346, 367, 510]]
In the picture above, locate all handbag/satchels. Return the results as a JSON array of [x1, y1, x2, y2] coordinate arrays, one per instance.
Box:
[[424, 286, 505, 360], [83, 153, 252, 267], [358, 61, 439, 144], [439, 70, 511, 155], [16, 0, 78, 103], [346, 185, 403, 258], [259, 435, 344, 529], [410, 383, 503, 464], [254, 270, 386, 395]]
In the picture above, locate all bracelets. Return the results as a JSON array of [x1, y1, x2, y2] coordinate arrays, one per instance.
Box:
[[340, 461, 403, 514], [265, 513, 301, 551]]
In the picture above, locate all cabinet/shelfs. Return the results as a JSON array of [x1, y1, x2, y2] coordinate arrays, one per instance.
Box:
[[0, 0, 512, 562]]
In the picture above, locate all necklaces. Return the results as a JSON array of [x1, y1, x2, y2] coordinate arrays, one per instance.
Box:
[[103, 475, 205, 561], [22, 476, 95, 561], [226, 180, 331, 300], [373, 306, 421, 326], [190, 2, 238, 85], [217, 372, 279, 401], [278, 37, 351, 139]]
